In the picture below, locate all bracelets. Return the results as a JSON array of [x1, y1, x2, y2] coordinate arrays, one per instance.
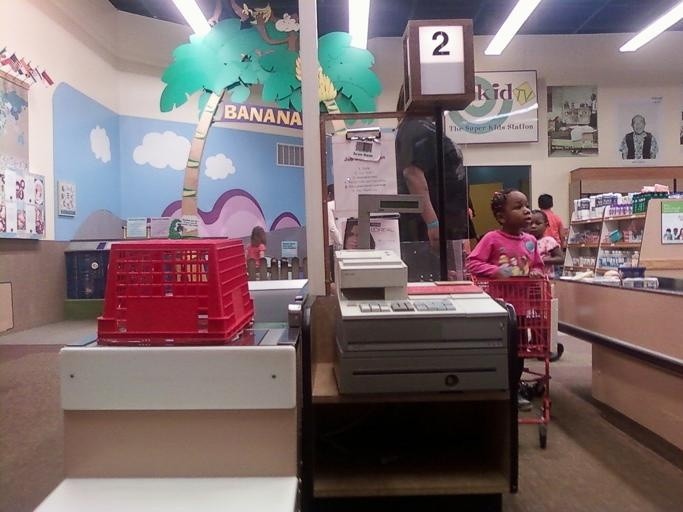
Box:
[[427, 220, 439, 228]]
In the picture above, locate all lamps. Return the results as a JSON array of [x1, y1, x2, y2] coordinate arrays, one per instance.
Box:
[[347, 0, 371, 51], [618, 1, 683, 54], [400, 16, 476, 115], [484, 0, 542, 56], [174, 0, 214, 36]]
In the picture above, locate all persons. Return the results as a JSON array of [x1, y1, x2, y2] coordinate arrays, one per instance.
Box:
[[467, 197, 479, 250], [664, 229, 672, 240], [537, 193, 564, 277], [245, 225, 268, 277], [326, 183, 340, 283], [524, 209, 564, 362], [588, 93, 597, 130], [396, 80, 468, 283], [678, 228, 683, 240], [672, 228, 679, 240], [343, 217, 374, 249], [463, 186, 547, 413], [554, 116, 572, 132], [619, 113, 659, 160]]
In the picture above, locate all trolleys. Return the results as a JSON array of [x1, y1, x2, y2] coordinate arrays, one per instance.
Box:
[[472, 272, 553, 451]]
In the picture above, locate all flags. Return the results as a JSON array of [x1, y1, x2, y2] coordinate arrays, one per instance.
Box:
[[0, 47, 54, 89]]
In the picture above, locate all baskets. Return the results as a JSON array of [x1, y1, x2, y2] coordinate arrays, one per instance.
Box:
[[96, 234, 255, 348]]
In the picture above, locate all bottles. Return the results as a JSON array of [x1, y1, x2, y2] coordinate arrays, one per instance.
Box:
[[632, 191, 668, 213], [609, 203, 633, 216], [599, 250, 639, 268]]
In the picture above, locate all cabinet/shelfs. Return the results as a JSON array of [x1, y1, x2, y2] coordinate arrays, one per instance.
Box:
[[30, 283, 310, 512], [563, 164, 683, 452], [303, 296, 521, 510]]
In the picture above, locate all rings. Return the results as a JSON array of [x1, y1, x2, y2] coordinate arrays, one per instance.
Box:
[[433, 238, 438, 241]]
